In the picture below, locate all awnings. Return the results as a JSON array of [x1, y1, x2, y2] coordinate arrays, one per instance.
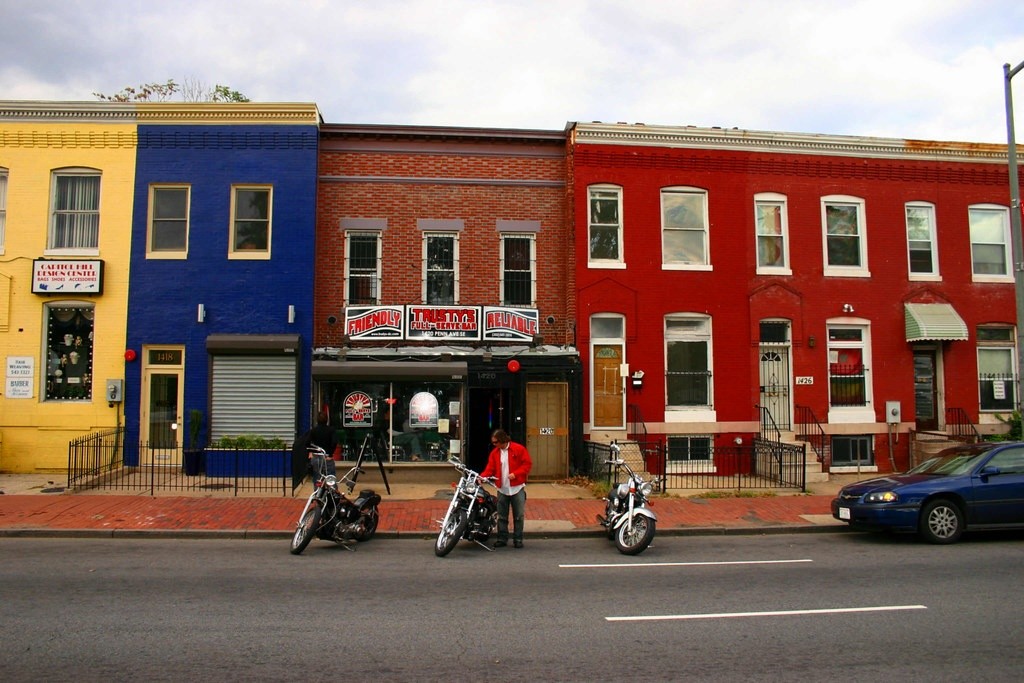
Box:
[[904, 303, 969, 342]]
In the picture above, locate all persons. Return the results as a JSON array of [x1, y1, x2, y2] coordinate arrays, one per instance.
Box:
[[380, 407, 425, 461], [304, 412, 339, 498], [478, 429, 532, 548]]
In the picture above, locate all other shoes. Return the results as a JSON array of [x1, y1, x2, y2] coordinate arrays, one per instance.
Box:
[[493, 541, 507, 547], [513, 539, 523, 549]]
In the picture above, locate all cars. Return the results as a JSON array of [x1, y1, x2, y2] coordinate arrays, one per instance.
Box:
[[831, 440, 1024, 545]]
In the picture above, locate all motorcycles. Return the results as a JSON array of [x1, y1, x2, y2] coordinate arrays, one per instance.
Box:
[[435, 454, 499, 558], [289, 442, 381, 556], [595, 444, 660, 556]]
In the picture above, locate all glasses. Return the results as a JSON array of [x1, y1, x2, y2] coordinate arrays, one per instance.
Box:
[[492, 439, 499, 444]]
